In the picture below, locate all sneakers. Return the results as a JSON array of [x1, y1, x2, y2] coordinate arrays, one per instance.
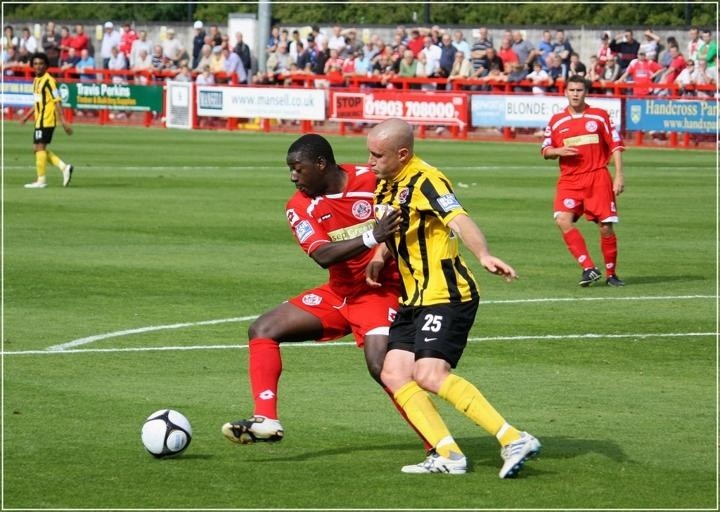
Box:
[[605, 273, 627, 288], [219, 412, 286, 447], [495, 430, 543, 480], [23, 180, 50, 189], [399, 448, 469, 477], [61, 163, 74, 187], [577, 266, 604, 287]]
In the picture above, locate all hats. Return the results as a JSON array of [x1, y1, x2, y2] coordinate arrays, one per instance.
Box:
[[213, 45, 223, 53], [637, 48, 647, 55], [193, 20, 204, 29], [166, 28, 175, 34], [104, 21, 113, 28]]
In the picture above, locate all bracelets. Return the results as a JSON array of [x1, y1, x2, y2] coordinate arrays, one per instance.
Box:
[[362, 229, 378, 249]]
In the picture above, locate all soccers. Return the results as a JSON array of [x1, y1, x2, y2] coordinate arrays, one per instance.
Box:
[[141, 409, 191, 458]]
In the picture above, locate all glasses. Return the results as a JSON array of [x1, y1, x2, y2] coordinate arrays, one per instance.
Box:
[[686, 63, 695, 66]]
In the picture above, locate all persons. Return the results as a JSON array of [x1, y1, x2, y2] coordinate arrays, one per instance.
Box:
[[19, 52, 74, 190], [366, 116, 542, 478], [221, 134, 437, 460], [2, 19, 719, 100], [540, 74, 627, 290]]
